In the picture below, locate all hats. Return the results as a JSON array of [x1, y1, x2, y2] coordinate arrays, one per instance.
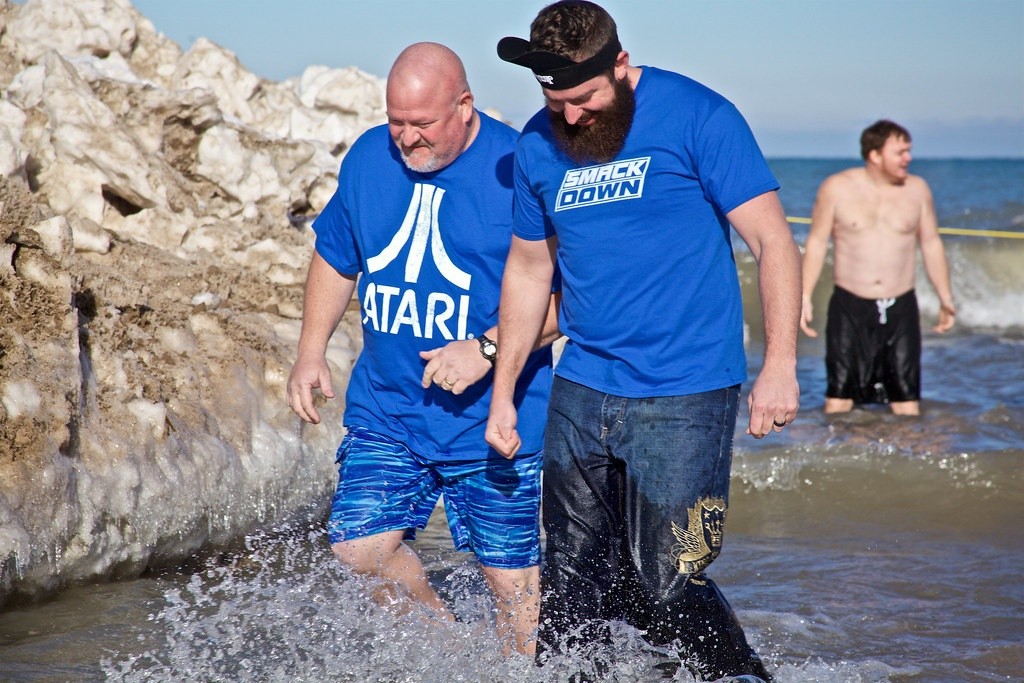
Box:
[[498, 26, 622, 90]]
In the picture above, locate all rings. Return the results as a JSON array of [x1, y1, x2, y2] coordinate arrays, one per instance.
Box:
[[444, 377, 453, 387], [774, 420, 786, 428]]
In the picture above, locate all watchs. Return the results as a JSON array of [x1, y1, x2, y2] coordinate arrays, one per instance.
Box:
[[477, 335, 498, 366]]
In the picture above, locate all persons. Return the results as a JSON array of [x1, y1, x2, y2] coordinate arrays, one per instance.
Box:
[[287, 41, 568, 661], [485, 0, 801, 682], [799, 118, 959, 418]]
